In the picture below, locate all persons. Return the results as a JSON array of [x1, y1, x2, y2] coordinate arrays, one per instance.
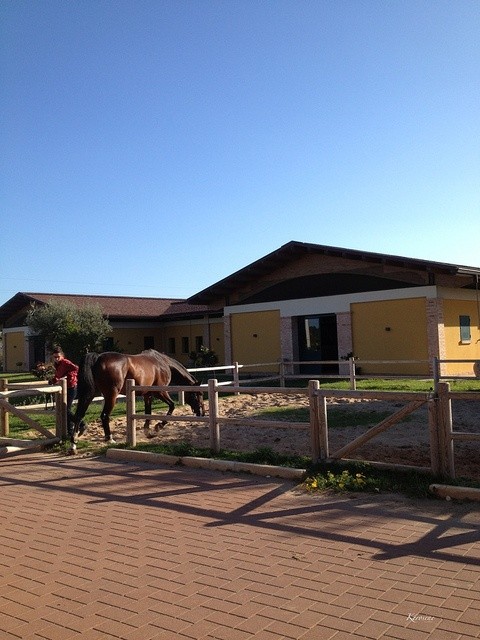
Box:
[[52, 346, 88, 438]]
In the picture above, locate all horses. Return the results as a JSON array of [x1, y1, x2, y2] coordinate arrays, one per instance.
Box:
[[66, 349, 208, 455]]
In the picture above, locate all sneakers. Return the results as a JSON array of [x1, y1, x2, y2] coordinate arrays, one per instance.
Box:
[[78, 423, 89, 437]]
